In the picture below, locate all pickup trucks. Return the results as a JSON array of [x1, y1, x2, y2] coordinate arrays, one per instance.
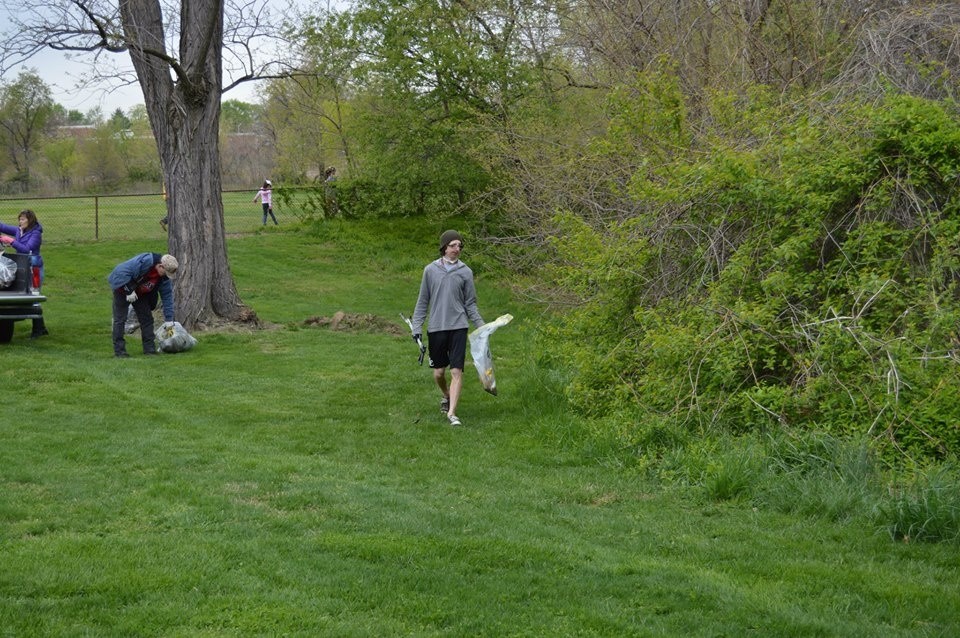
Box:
[[0, 250, 50, 344]]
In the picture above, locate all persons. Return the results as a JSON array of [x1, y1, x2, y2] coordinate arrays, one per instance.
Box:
[[108, 252, 179, 358], [0, 210, 48, 339], [326, 167, 337, 181], [159, 181, 168, 232], [411, 230, 484, 426], [254, 180, 278, 225]]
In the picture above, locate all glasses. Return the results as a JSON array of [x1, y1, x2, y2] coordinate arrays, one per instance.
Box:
[[447, 244, 463, 248]]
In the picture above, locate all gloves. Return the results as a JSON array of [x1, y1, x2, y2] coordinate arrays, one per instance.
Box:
[[126, 292, 137, 302], [163, 321, 174, 330], [412, 334, 422, 343]]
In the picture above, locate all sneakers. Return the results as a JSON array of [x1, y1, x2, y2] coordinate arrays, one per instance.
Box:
[[441, 395, 450, 413], [447, 415, 463, 426]]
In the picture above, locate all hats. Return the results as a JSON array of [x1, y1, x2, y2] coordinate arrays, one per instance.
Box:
[[264, 180, 272, 189], [160, 254, 179, 279], [439, 230, 462, 255]]
